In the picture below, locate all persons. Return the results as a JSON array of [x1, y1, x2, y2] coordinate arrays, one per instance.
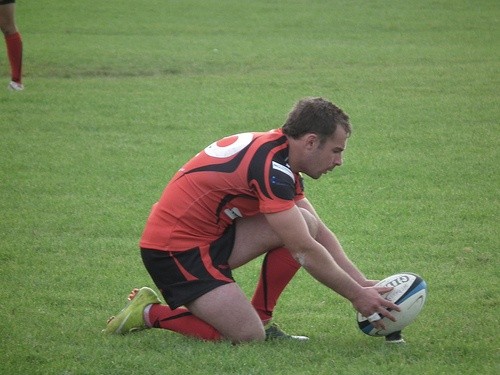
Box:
[[0, 0, 24, 91], [101, 98, 403, 344]]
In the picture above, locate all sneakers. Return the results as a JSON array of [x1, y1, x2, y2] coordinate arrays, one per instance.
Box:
[[263, 320, 310, 343], [103, 286, 162, 336]]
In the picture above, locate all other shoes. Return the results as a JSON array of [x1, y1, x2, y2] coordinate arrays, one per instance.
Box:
[[7, 80, 24, 91]]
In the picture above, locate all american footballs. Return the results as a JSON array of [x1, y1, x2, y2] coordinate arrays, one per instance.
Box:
[[357, 272, 426, 337]]
